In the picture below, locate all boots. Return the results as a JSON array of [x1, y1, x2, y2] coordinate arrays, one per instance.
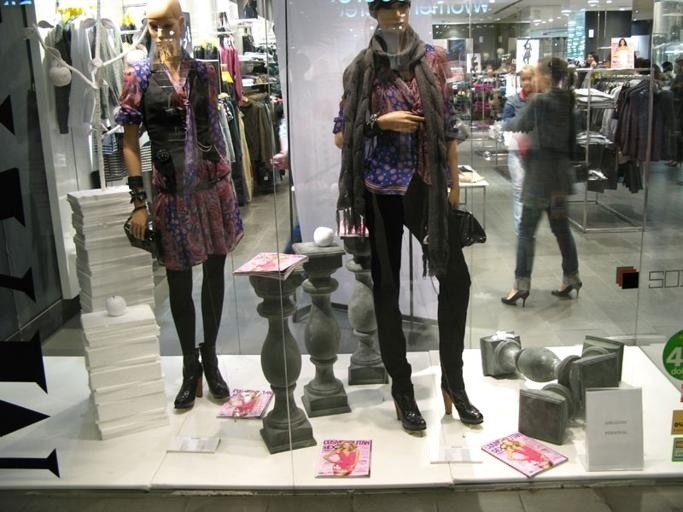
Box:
[[199, 343, 230, 403], [174, 349, 202, 411]]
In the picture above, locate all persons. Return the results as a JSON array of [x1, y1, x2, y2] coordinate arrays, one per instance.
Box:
[[270, 119, 301, 255], [663, 58, 682, 168], [501, 63, 536, 238], [497, 55, 582, 305], [612, 39, 630, 58], [522, 39, 532, 64], [488, 47, 682, 102], [114, 0, 246, 410], [330, 0, 486, 433]]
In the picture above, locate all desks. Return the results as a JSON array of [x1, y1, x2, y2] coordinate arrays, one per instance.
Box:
[[453, 165, 488, 236]]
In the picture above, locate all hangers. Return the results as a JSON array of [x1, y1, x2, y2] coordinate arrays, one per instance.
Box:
[[580, 71, 652, 95], [194, 28, 285, 118]]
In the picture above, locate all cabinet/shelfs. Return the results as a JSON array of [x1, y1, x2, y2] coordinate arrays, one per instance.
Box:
[[569, 67, 654, 231]]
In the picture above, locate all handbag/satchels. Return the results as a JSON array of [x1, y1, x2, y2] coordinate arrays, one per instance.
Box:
[[455, 208, 486, 246], [124, 216, 162, 262]]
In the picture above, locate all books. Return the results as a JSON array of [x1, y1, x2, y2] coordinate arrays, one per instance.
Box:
[[231, 246, 308, 285]]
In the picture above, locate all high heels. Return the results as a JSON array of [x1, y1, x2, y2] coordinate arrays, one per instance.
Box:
[[391, 384, 426, 431], [552, 281, 582, 296], [501, 290, 529, 307], [441, 372, 483, 425]]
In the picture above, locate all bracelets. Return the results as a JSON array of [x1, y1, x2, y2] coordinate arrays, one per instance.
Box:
[[362, 111, 381, 139], [131, 205, 146, 213], [126, 176, 147, 204]]
